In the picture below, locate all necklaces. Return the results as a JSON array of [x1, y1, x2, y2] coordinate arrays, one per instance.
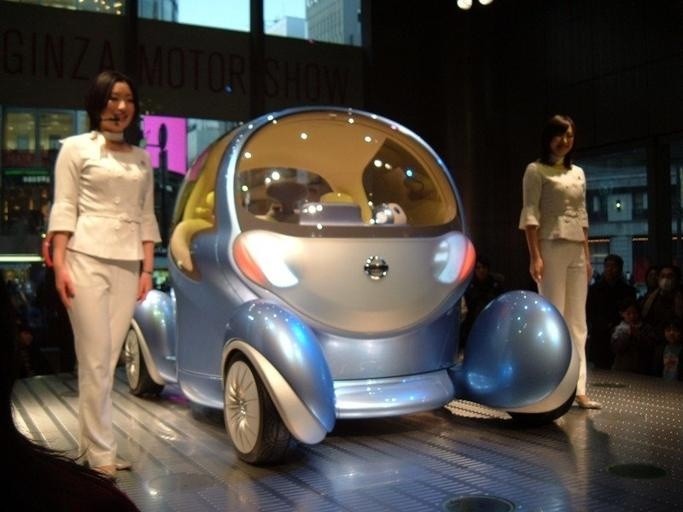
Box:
[[100, 129, 124, 143], [548, 153, 564, 166]]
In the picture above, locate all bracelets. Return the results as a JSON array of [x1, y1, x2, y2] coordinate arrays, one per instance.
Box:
[[141, 270, 152, 276]]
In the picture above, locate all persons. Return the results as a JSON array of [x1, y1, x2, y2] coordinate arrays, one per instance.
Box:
[[517, 112, 603, 410], [458, 253, 681, 381], [0, 265, 142, 511], [46, 67, 166, 482]]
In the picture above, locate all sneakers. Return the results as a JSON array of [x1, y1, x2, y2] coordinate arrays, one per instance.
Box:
[[89, 464, 115, 481], [114, 455, 133, 470], [574, 394, 605, 410]]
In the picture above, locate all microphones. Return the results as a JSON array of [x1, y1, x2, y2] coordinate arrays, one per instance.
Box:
[[101, 117, 122, 122]]
